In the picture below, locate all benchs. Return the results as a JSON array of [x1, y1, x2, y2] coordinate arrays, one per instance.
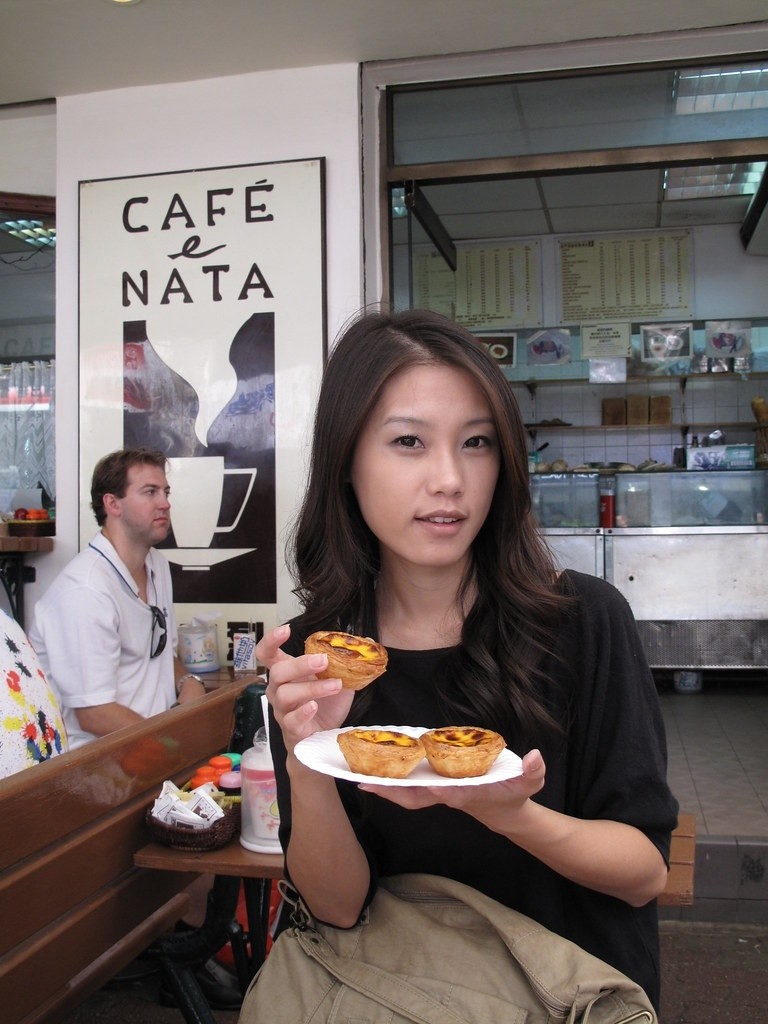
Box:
[[0, 677, 266, 1024]]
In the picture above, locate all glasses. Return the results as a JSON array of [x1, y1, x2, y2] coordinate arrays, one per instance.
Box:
[[150, 604, 167, 658]]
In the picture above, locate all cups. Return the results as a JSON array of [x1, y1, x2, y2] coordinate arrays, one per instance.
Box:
[[648, 343, 666, 357], [176, 625, 220, 673]]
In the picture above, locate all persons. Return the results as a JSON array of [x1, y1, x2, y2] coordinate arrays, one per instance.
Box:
[[0, 609, 69, 779], [256, 308, 679, 1017], [29, 444, 207, 751]]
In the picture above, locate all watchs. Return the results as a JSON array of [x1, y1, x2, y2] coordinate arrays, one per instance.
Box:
[[176, 673, 205, 691]]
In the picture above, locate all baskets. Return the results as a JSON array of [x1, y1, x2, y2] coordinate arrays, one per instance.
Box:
[[146, 795, 241, 853]]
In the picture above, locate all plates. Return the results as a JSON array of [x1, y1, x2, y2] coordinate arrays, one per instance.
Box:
[[664, 335, 683, 350], [584, 458, 625, 469], [488, 344, 508, 358], [707, 330, 736, 353], [292, 723, 521, 788]]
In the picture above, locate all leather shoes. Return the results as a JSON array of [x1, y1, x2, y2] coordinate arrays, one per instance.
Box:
[[160, 955, 247, 1009]]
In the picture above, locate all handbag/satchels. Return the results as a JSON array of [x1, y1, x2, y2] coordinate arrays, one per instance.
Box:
[[237, 874, 659, 1024]]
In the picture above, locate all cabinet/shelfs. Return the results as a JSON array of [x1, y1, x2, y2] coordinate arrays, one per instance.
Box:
[[509, 371, 768, 432]]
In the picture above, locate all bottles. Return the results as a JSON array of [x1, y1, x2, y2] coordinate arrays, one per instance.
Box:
[[190, 752, 241, 796], [597, 488, 617, 528], [14, 508, 50, 521], [691, 434, 700, 448]]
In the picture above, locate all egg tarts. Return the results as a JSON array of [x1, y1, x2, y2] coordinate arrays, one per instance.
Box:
[[337, 729, 425, 777], [303, 633, 388, 689], [420, 725, 505, 777]]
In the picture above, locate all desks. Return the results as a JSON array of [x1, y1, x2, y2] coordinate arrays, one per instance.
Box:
[[133, 812, 697, 998], [198, 666, 265, 693], [0, 537, 53, 630]]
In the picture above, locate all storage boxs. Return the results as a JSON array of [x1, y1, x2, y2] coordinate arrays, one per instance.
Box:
[[234, 632, 257, 671], [687, 443, 756, 471]]
